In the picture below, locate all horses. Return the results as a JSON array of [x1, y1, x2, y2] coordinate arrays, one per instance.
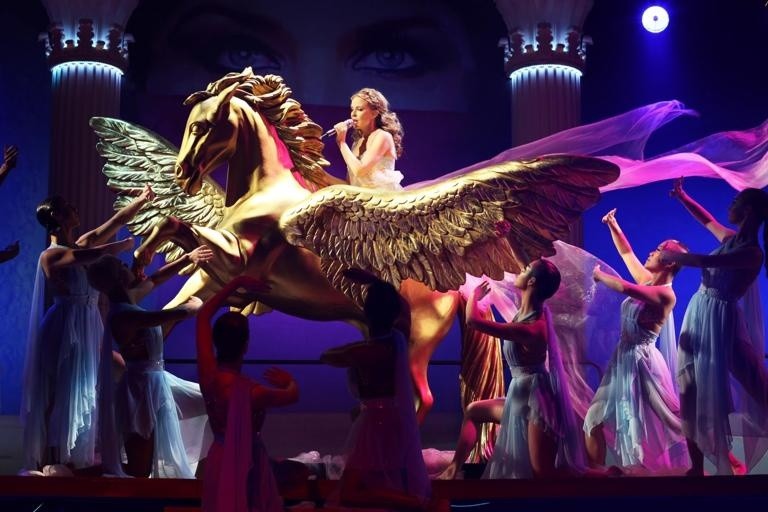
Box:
[[86, 65, 621, 430]]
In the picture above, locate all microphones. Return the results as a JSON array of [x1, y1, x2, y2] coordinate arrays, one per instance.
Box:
[[320, 119, 353, 140]]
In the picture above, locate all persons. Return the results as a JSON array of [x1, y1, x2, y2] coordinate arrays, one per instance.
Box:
[[196, 275, 317, 511], [18, 181, 156, 482], [334, 88, 405, 193], [0, 146, 23, 263], [317, 265, 430, 512], [581, 207, 749, 474], [85, 245, 215, 477], [669, 175, 767, 477], [426, 258, 627, 480]]
